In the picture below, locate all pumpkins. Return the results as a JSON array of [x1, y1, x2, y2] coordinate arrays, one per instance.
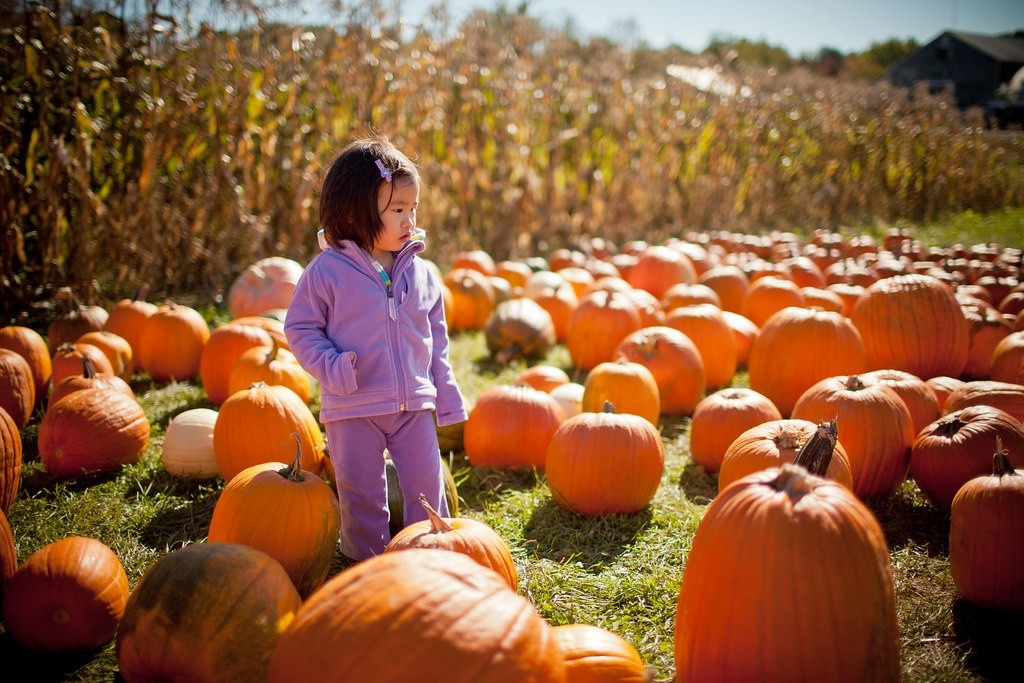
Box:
[[0, 229, 1024, 683]]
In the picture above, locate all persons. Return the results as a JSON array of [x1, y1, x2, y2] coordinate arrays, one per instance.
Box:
[[284, 139, 468, 564]]
